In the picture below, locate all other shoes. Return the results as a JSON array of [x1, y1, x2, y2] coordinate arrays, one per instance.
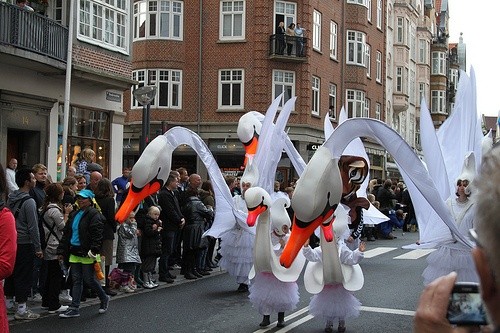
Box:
[[79, 259, 220, 302], [59, 290, 73, 302], [324, 327, 333, 333], [360, 226, 408, 241], [260, 320, 271, 326], [276, 321, 285, 328], [338, 326, 346, 333], [33, 293, 42, 303]]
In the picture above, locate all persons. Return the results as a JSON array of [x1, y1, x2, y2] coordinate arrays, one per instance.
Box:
[[0, 164, 17, 333], [248, 224, 299, 327], [413, 145, 500, 333], [0, 148, 418, 319], [303, 232, 366, 333], [276, 22, 305, 57], [14, 0, 48, 16]]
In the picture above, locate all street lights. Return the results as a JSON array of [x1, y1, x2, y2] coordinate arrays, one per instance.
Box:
[[132, 84, 159, 152]]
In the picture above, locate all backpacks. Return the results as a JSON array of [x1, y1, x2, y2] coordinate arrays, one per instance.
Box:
[[182, 197, 201, 226], [38, 204, 61, 252]]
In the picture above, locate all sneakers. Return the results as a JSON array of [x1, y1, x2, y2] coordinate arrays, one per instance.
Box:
[[40, 305, 49, 309], [58, 308, 80, 317], [99, 296, 110, 313], [15, 309, 40, 320], [6, 305, 18, 314], [48, 304, 69, 313]]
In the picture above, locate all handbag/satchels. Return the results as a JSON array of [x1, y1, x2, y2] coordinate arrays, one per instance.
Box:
[[94, 257, 106, 286], [108, 268, 135, 292]]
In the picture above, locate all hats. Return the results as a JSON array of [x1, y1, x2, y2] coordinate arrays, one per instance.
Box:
[[272, 197, 291, 237], [241, 156, 255, 191], [76, 189, 95, 199], [455, 151, 476, 198], [87, 163, 103, 171]]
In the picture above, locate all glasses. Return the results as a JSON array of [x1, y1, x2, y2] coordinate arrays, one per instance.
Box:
[[469, 227, 486, 250]]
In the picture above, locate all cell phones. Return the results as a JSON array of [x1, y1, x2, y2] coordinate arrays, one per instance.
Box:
[[444, 281, 489, 325]]
